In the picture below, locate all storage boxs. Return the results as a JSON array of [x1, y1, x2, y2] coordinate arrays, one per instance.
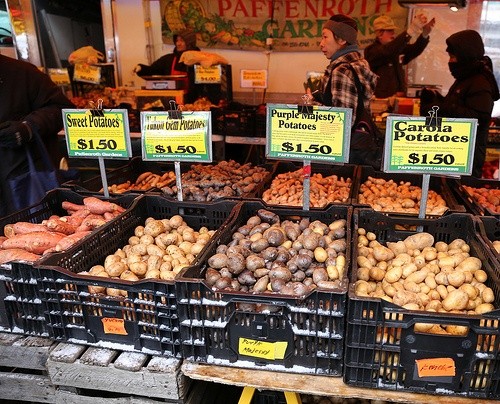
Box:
[[0, 161, 500, 404]]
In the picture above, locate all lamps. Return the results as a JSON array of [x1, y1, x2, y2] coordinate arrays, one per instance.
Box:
[[449, 0, 467, 12]]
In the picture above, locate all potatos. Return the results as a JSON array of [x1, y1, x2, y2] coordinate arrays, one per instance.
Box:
[[459, 185, 500, 215], [66, 90, 127, 108], [177, 96, 218, 112], [194, 209, 346, 355], [357, 176, 449, 216], [0, 197, 126, 265], [62, 215, 218, 328], [97, 157, 271, 203], [356, 228, 500, 389], [262, 168, 351, 209]]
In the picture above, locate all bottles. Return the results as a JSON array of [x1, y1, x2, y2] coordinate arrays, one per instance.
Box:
[[107, 48, 114, 62]]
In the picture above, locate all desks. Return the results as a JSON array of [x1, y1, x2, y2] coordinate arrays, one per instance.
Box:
[[57, 131, 223, 159], [226, 136, 267, 164]]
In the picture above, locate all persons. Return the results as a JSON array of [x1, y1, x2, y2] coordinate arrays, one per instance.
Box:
[[419, 30, 500, 179], [305, 13, 379, 130], [0, 54, 77, 178], [363, 15, 435, 98], [133, 30, 202, 104]]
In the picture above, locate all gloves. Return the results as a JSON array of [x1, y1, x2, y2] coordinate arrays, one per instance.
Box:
[[0, 121, 29, 147]]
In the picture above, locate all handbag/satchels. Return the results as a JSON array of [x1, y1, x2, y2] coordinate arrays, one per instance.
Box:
[[351, 113, 385, 169], [10, 123, 64, 224]]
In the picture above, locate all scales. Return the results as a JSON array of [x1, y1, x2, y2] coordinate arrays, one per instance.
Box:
[[142, 75, 188, 90], [407, 84, 443, 98]]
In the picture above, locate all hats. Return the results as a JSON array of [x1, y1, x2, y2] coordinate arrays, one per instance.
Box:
[[373, 16, 399, 30]]
